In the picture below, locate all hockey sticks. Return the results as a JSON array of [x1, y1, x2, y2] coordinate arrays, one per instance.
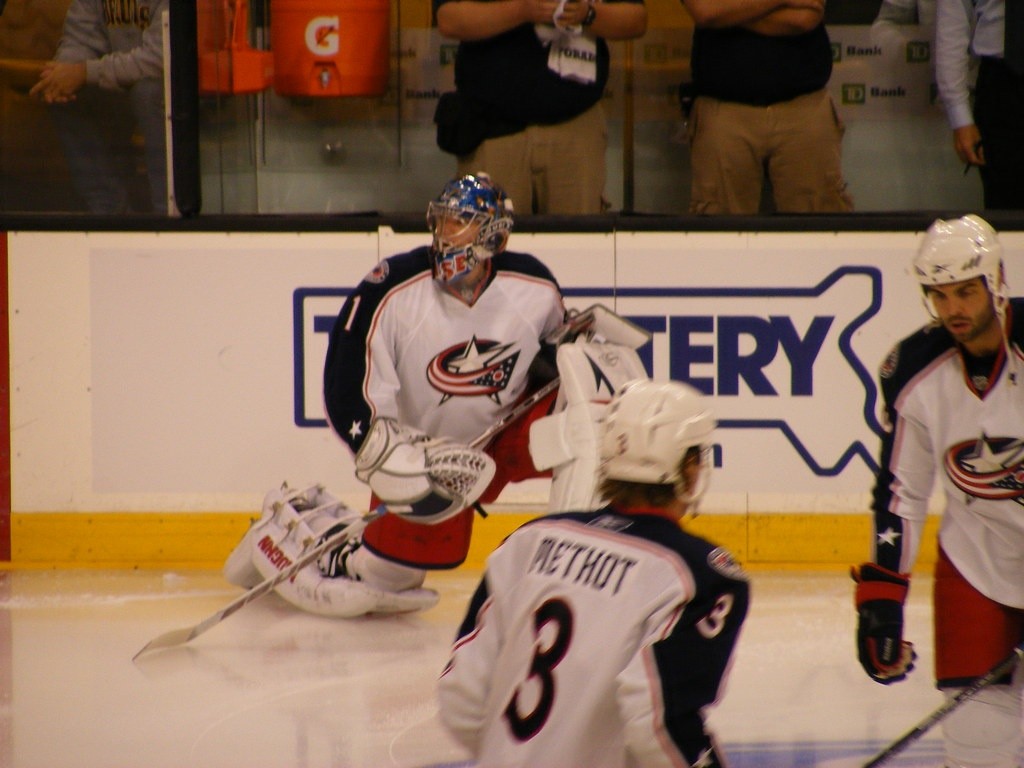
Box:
[[132, 373, 563, 660], [862, 638, 1024, 768]]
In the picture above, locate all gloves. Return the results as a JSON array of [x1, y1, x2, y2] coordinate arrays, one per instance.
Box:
[[850, 562, 917, 684]]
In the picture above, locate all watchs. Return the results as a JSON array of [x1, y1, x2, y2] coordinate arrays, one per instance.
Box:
[[582, 5, 596, 25]]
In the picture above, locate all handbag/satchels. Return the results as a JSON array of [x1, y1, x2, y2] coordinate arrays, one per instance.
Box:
[[432, 92, 483, 156]]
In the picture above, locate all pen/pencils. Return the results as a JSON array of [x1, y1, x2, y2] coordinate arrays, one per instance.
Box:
[[963, 140, 987, 176]]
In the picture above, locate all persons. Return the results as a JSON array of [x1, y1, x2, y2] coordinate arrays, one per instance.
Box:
[[936, 0, 1023, 228], [850, 213, 1024, 768], [221, 170, 648, 620], [30, 0, 169, 214], [438, 378, 751, 768], [434, 0, 648, 217], [681, 0, 855, 213]]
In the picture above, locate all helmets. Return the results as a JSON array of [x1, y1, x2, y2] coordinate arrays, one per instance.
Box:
[[600, 377, 714, 505], [426, 173, 513, 282], [915, 214, 1004, 295]]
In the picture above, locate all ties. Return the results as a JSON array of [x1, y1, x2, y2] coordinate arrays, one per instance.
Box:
[[1004, 0, 1024, 75]]
